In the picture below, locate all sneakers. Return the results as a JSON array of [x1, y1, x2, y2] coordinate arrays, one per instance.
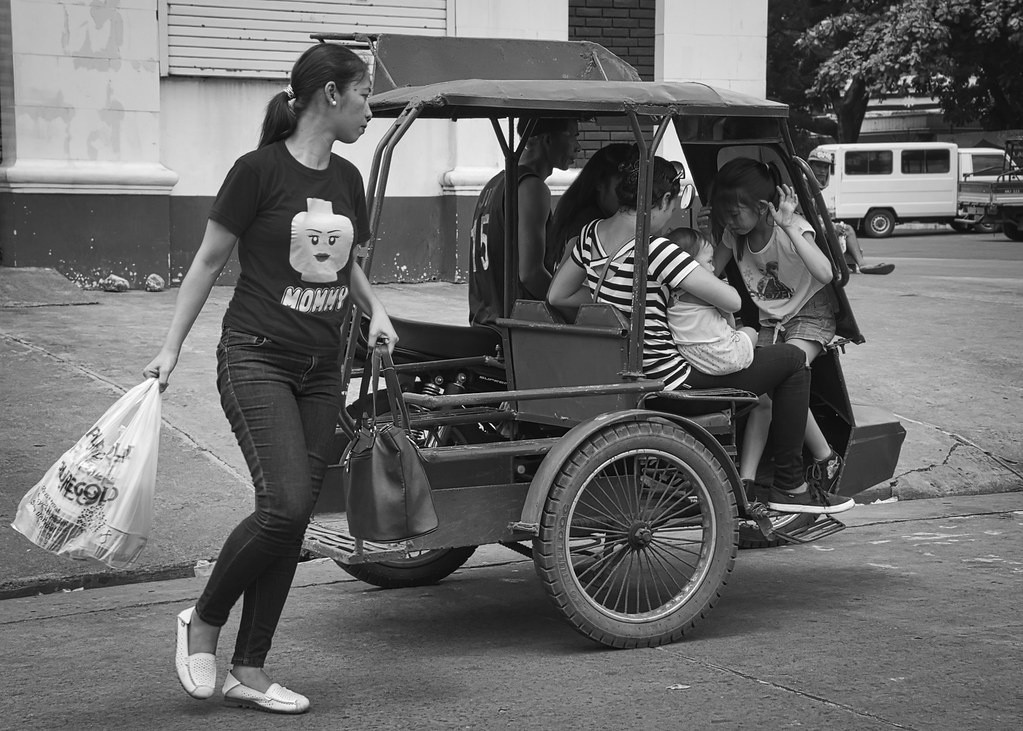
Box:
[[766, 465, 856, 513]]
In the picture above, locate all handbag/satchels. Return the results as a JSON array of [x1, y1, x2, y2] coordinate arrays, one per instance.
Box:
[[9, 377, 161, 573], [338, 344, 440, 543]]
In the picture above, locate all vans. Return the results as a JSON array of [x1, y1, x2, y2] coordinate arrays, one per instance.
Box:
[[810, 139, 1023, 238]]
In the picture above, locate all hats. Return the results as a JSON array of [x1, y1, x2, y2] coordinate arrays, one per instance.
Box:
[[807, 147, 834, 165]]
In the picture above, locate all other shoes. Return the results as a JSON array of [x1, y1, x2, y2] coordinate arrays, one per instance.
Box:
[[221, 669, 310, 715], [741, 478, 758, 509], [175, 606, 221, 699], [812, 444, 843, 493]]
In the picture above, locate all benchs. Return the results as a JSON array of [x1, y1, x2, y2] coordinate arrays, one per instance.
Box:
[[508, 298, 759, 436]]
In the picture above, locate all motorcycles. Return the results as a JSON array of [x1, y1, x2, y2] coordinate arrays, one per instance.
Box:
[[293, 29, 906, 653]]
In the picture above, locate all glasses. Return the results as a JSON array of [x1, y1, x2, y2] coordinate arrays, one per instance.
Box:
[[670, 161, 685, 185]]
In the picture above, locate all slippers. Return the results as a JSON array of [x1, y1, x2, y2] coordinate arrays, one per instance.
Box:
[[863, 263, 895, 275]]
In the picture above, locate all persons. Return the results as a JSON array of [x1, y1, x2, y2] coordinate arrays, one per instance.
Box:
[[468, 118, 895, 513], [142, 43, 399, 714]]
